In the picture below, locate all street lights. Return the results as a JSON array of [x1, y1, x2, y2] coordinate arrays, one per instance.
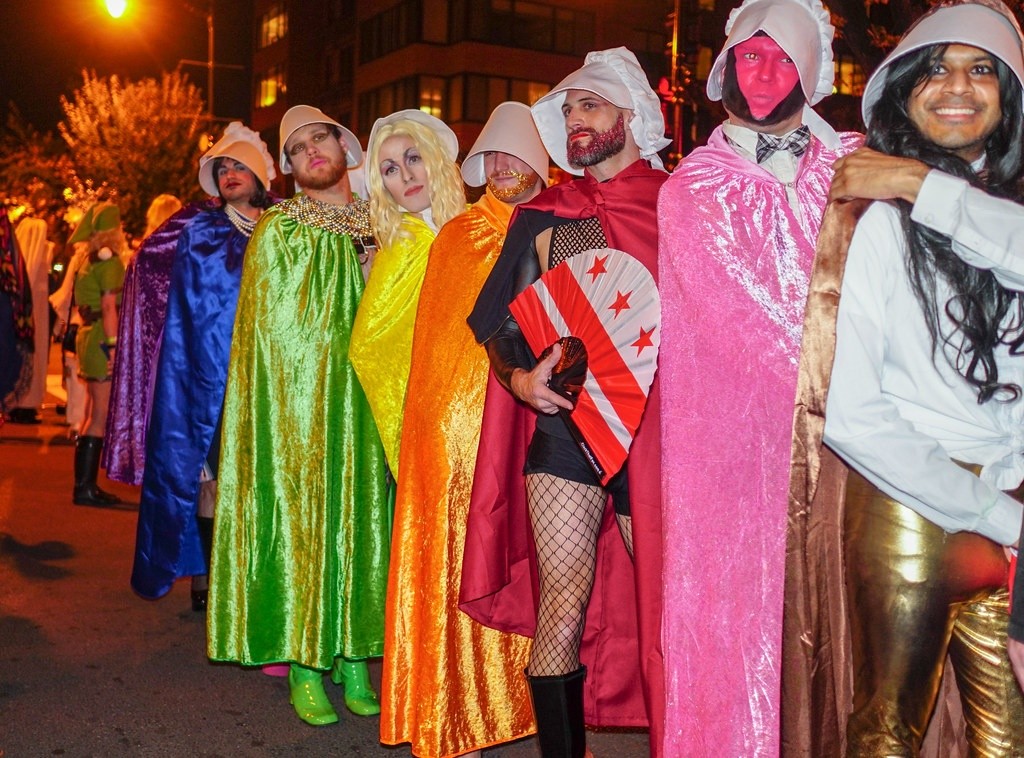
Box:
[[106, 0, 215, 139]]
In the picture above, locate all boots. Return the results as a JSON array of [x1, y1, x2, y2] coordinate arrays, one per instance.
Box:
[[73, 434, 123, 506], [524, 662, 587, 758]]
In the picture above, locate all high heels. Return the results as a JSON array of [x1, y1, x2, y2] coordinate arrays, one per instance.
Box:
[[289, 662, 339, 726], [332, 656, 379, 715]]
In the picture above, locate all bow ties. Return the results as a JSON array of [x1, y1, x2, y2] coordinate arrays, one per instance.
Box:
[[756, 125, 810, 164]]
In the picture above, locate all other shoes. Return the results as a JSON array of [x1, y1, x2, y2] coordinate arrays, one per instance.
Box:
[[10, 408, 42, 423], [191, 587, 208, 607]]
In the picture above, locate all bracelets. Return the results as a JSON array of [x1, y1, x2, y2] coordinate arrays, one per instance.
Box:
[[1009, 546, 1020, 556], [104, 336, 117, 344]]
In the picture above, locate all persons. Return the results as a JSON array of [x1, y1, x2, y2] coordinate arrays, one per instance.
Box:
[[0, 204, 56, 424], [780, 1, 1024, 758], [100, 131, 290, 622], [203, 104, 396, 727], [63, 198, 129, 506], [657, 1, 871, 758], [465, 45, 677, 758], [380, 101, 561, 758], [350, 105, 477, 487]]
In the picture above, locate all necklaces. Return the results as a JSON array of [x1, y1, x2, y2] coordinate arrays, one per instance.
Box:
[[223, 203, 256, 238]]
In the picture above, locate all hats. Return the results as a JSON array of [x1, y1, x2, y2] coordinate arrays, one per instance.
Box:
[[366, 110, 458, 197], [281, 105, 362, 175], [529, 45, 673, 175], [861, 0, 1024, 154], [66, 201, 122, 244], [460, 101, 549, 189], [706, 0, 836, 106], [198, 122, 277, 197]]
